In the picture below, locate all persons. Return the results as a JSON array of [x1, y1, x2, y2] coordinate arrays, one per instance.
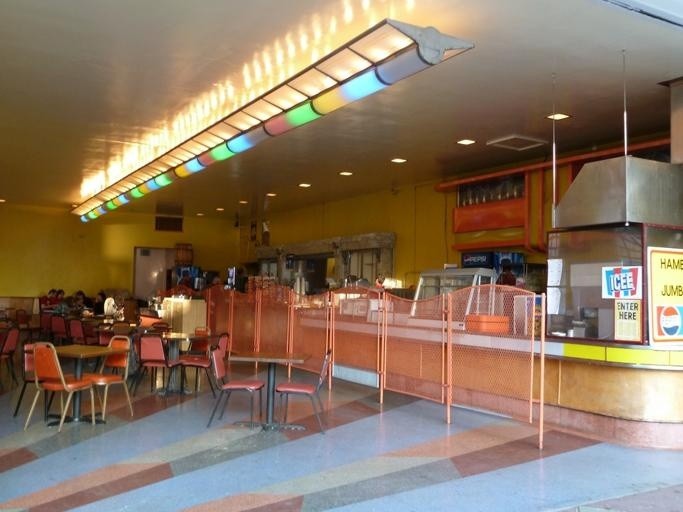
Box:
[[179, 271, 191, 289], [496, 259, 516, 287], [39, 289, 106, 315], [235, 268, 247, 293]]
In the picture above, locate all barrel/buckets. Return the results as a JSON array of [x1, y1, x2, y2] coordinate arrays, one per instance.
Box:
[[194, 277, 204, 290]]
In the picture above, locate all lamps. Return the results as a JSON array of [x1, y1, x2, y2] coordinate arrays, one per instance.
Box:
[[69, 18, 474, 224]]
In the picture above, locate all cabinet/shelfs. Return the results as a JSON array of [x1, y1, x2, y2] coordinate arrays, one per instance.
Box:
[[433, 164, 549, 254], [550, 137, 670, 207]]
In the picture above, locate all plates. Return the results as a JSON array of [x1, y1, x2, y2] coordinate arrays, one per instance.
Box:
[[552, 332, 566, 336]]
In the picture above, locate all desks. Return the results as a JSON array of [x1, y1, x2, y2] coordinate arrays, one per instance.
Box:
[[227, 352, 314, 433], [45, 344, 132, 428]]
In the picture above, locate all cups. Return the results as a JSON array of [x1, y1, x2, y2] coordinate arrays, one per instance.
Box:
[[567, 329, 573, 337]]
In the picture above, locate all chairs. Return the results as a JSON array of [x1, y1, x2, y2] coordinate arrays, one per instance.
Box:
[[80, 334, 133, 421], [208, 344, 266, 432], [0, 297, 230, 397], [276, 350, 333, 433], [10, 339, 55, 422], [23, 341, 95, 430]]
